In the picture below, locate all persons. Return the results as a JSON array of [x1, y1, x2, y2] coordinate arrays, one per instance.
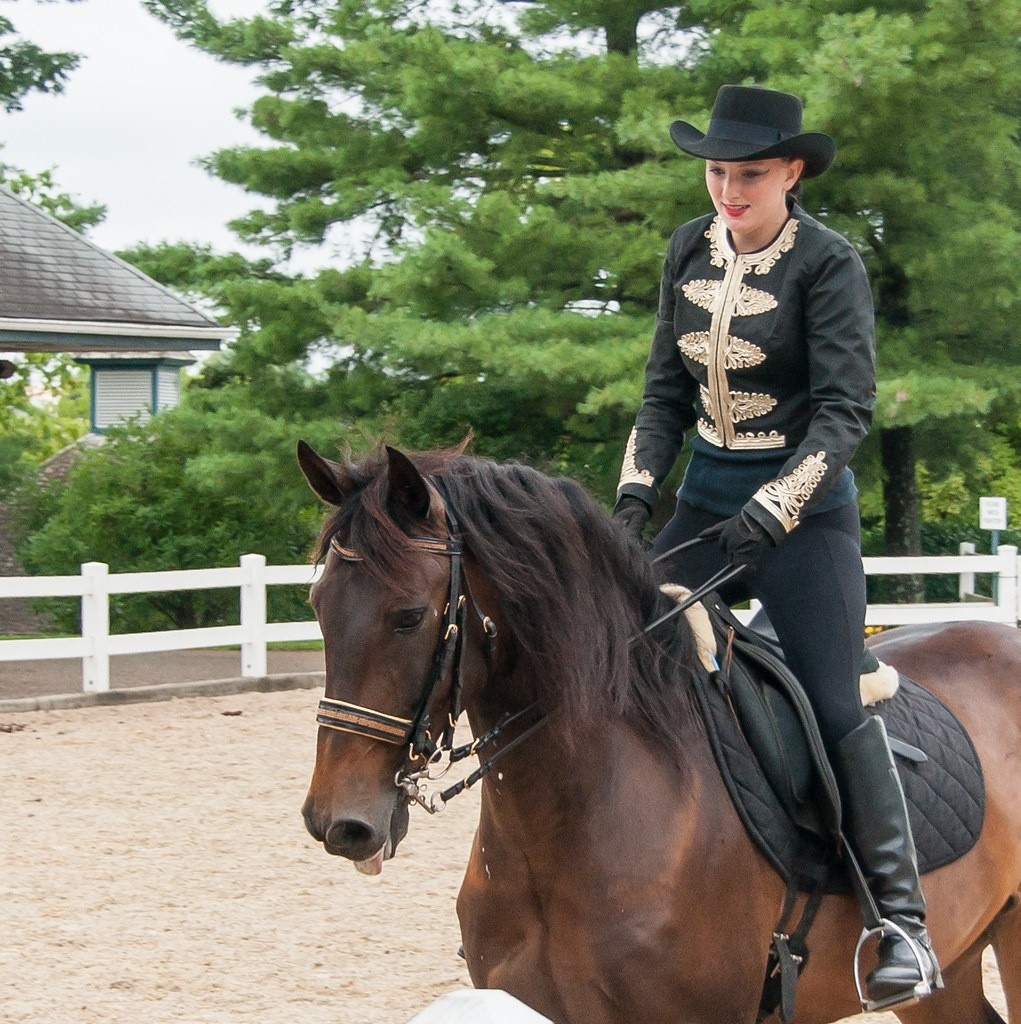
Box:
[[613, 82, 946, 1001]]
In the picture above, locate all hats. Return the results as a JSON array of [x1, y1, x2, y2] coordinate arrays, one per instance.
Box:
[[671, 84, 836, 180]]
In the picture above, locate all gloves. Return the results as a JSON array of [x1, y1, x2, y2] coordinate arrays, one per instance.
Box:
[[699, 513, 771, 577], [611, 498, 650, 536]]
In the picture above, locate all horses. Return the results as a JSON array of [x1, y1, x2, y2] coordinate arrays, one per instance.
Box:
[[296, 423, 1020, 1022]]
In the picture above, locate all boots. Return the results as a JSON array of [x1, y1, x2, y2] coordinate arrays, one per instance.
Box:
[[830, 714, 945, 1001]]
